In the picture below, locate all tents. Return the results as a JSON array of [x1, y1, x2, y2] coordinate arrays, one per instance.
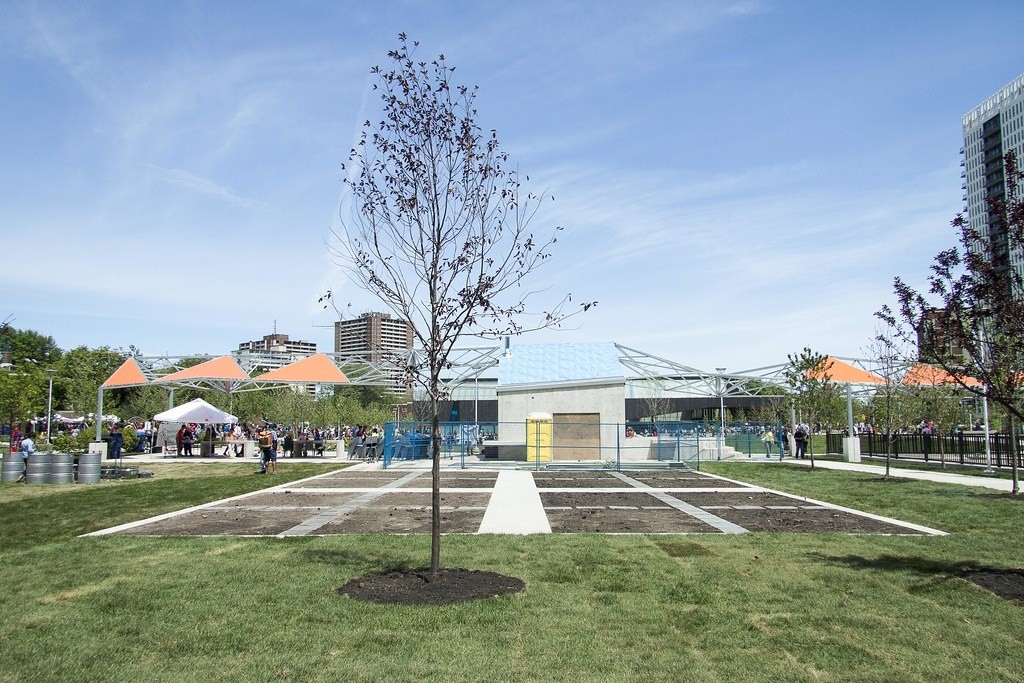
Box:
[[154, 399, 239, 457], [25, 411, 121, 430]]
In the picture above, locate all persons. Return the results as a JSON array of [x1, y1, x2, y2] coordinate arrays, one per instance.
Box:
[[779, 426, 790, 454], [19, 433, 37, 477], [760, 427, 775, 458], [175, 420, 498, 458], [626, 423, 779, 437], [11, 425, 152, 459], [811, 420, 959, 437], [973, 420, 984, 431], [792, 424, 808, 459]]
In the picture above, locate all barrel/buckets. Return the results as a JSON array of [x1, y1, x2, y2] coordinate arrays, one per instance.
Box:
[[77, 453, 102, 484], [26, 455, 51, 484], [50, 453, 74, 483], [2, 452, 24, 482]]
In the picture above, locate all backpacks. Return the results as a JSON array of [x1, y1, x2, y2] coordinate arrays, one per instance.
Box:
[[259, 432, 272, 448]]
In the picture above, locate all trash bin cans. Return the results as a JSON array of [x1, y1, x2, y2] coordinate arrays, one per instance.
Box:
[[92, 432, 123, 459], [405, 433, 431, 459], [136, 432, 146, 451]]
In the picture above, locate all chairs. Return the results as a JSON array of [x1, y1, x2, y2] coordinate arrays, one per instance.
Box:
[[313, 441, 326, 456], [281, 441, 293, 458]]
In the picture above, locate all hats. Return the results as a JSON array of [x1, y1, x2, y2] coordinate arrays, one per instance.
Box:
[[269, 424, 274, 428]]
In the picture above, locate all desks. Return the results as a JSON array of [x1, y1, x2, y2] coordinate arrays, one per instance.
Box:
[[293, 440, 345, 459], [201, 440, 255, 458]]
